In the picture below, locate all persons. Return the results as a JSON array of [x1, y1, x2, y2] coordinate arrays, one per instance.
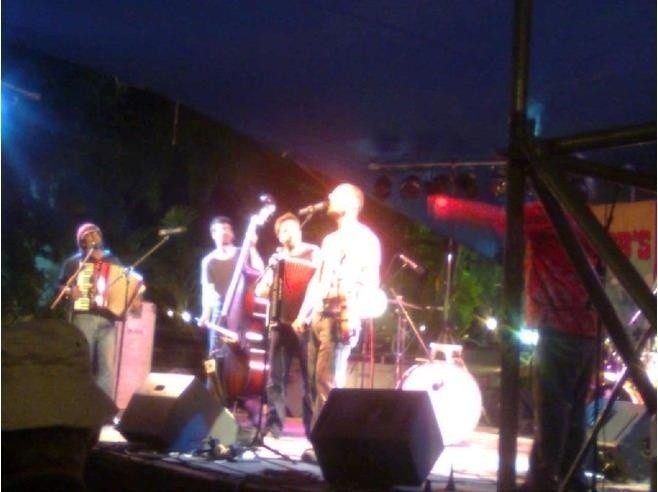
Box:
[[197, 216, 264, 410], [425, 168, 606, 490], [53, 223, 143, 400], [292, 181, 382, 464], [255, 211, 322, 444], [32, 245, 59, 274]]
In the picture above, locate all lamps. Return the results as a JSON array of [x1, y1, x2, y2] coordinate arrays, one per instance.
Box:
[[367, 160, 519, 199]]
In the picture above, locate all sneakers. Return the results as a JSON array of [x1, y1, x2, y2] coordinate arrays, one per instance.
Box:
[[302, 448, 317, 463]]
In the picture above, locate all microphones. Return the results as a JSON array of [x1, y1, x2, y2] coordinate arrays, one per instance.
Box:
[[298, 201, 328, 215], [399, 254, 425, 274], [158, 225, 187, 236]]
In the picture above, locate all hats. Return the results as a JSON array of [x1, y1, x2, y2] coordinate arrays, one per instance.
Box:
[[77, 222, 100, 242]]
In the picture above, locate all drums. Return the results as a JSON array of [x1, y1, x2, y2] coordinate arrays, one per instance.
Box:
[[393, 361, 483, 443]]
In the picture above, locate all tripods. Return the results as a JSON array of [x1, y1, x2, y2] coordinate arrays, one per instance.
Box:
[[226, 264, 301, 464], [432, 244, 491, 425]]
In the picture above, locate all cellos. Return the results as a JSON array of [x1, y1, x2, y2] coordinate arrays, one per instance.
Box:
[[216, 193, 277, 397]]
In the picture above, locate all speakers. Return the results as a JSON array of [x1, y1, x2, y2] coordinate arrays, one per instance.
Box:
[[114, 301, 157, 411], [114, 372, 239, 452], [308, 388, 446, 487], [584, 398, 657, 483]]
